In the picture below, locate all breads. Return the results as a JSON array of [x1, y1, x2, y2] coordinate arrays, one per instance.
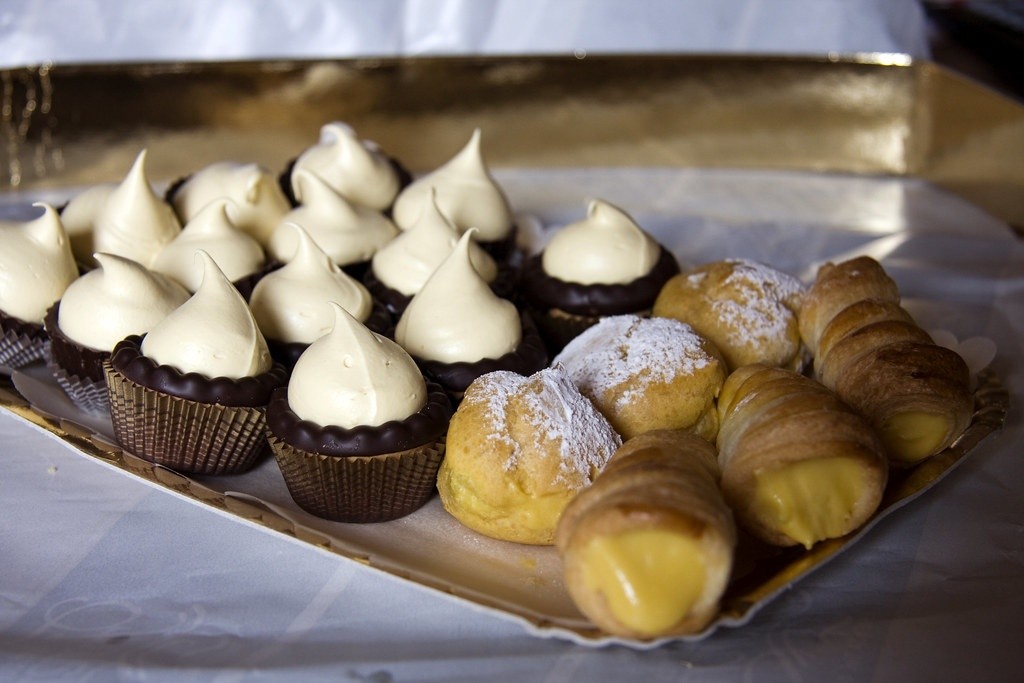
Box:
[[437, 255, 976, 642]]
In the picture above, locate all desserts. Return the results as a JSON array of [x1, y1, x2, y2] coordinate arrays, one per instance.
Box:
[[0, 118, 687, 526]]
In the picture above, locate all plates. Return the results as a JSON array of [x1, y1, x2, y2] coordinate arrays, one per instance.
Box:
[[0, 369, 1024, 652]]
